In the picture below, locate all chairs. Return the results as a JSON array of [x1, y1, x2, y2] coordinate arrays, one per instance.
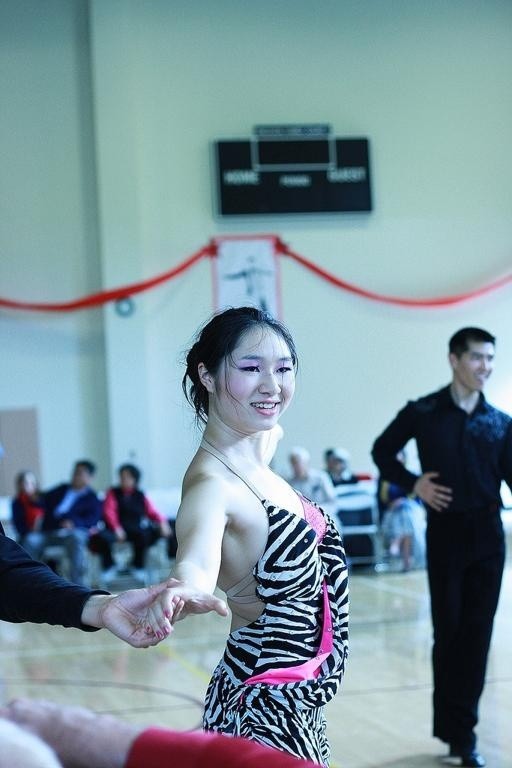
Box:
[[333, 482, 388, 572], [35, 512, 92, 583]]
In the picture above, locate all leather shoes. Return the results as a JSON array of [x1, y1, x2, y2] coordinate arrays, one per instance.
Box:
[[446, 737, 485, 768]]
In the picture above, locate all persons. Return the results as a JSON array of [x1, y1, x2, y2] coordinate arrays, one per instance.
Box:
[[1, 697, 324, 768], [371, 327, 512, 767], [142, 307, 349, 768], [286, 445, 428, 572], [12, 460, 172, 587], [0, 522, 185, 648]]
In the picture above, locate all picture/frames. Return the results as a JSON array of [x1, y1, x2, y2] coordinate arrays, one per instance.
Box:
[[212, 234, 282, 322]]
